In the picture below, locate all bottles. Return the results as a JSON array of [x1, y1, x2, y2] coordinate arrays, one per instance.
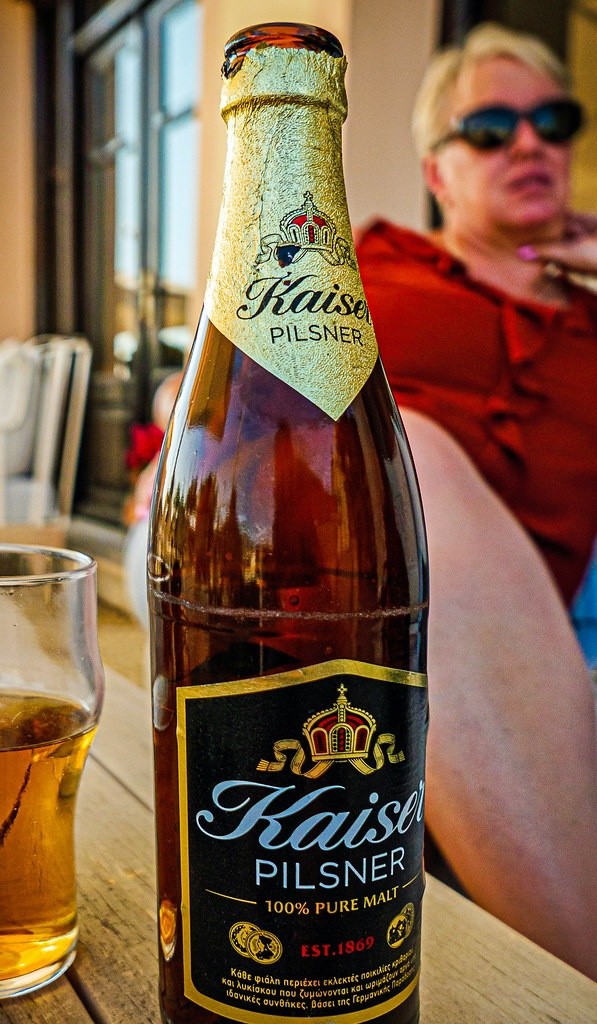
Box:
[[138, 17, 434, 1023]]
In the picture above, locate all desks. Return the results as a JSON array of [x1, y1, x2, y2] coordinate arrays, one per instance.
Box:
[[0, 657, 597, 1024]]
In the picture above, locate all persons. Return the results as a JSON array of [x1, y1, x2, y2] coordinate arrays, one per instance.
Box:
[[351, 26, 596, 975]]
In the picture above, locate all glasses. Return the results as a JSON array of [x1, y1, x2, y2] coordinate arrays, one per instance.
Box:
[[432, 97, 585, 151]]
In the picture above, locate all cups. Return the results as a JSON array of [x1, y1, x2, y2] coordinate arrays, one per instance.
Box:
[[1, 538, 113, 1005]]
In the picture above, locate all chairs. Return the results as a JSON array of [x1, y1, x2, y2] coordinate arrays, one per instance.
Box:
[[1, 331, 91, 598]]
